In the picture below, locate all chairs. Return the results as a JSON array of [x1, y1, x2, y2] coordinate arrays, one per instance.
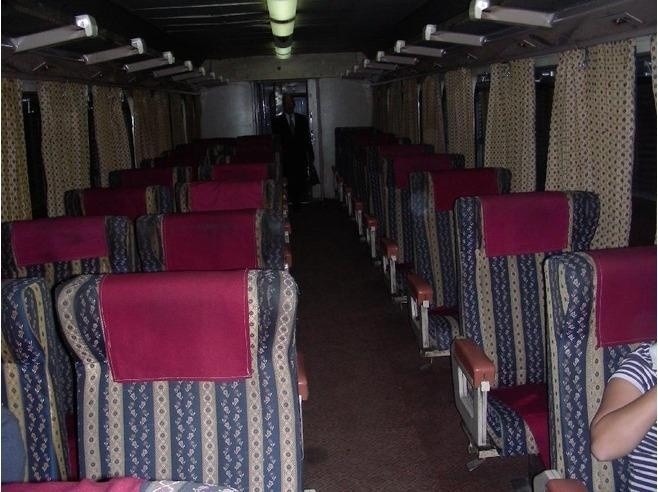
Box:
[[445, 186, 654, 492], [4, 133, 308, 491], [337, 124, 512, 356]]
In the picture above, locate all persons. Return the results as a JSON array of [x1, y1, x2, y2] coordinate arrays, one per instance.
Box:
[[270, 92, 316, 206], [589, 341, 657, 492]]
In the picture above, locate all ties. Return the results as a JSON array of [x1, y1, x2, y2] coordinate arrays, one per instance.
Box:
[[289, 115, 295, 135]]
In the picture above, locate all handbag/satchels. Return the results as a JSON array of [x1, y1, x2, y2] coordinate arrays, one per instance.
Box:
[[308, 162, 321, 187]]
